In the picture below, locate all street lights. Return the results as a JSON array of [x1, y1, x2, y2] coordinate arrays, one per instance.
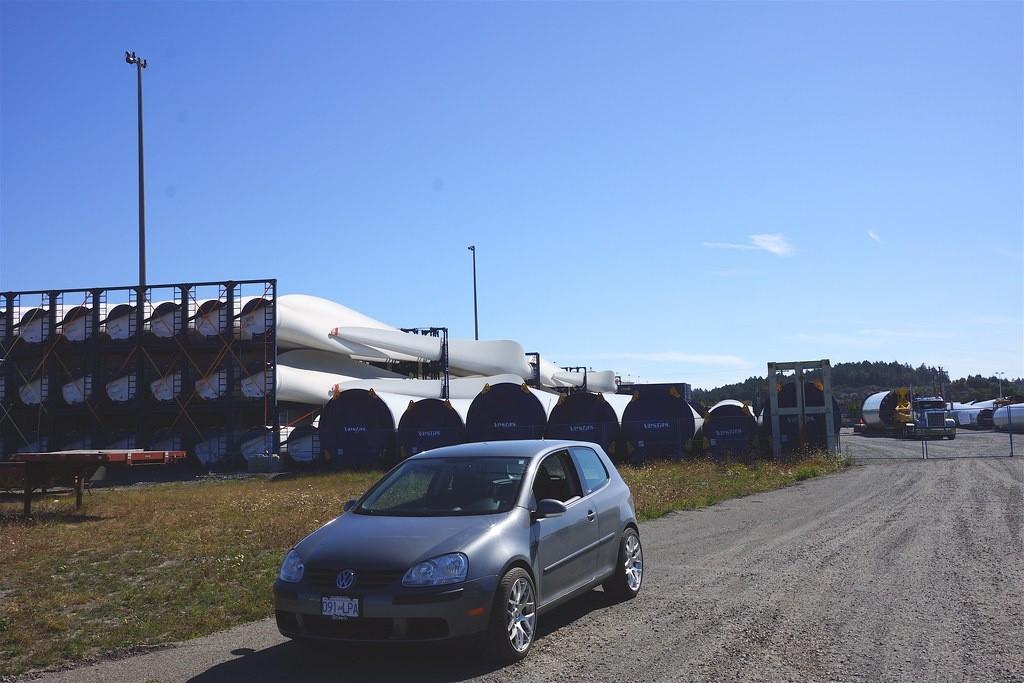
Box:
[[995, 372, 1005, 399], [468, 244, 479, 341], [125, 48, 147, 304]]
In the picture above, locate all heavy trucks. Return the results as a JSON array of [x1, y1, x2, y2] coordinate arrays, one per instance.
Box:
[[851, 381, 957, 440]]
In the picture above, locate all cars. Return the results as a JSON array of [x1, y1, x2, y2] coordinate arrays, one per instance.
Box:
[[271, 438, 644, 664]]
